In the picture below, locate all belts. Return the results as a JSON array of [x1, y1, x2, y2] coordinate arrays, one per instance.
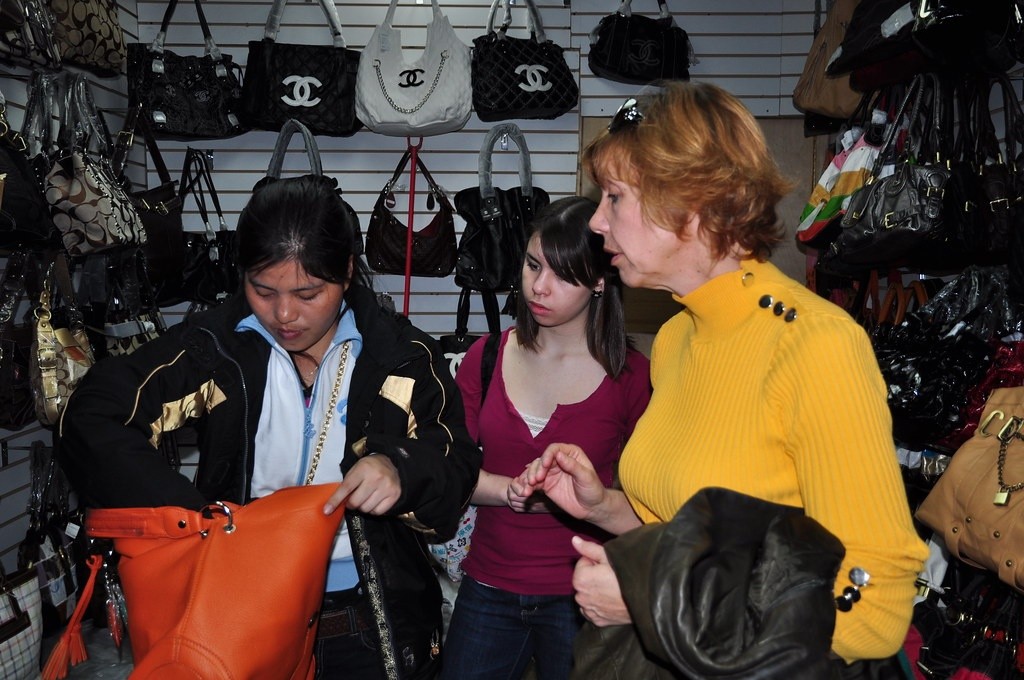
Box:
[[312, 605, 376, 640]]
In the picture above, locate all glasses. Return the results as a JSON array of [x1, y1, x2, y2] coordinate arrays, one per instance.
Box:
[[606, 98, 649, 136]]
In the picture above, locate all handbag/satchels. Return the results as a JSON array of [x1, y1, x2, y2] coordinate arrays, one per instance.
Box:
[[792, 0, 1024, 678], [0, 1, 694, 680]]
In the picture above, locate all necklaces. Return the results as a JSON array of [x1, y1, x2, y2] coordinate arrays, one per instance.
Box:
[[298, 356, 317, 381]]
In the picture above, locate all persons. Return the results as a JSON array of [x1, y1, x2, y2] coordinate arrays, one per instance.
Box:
[[431, 194, 654, 680], [52, 174, 483, 680], [519, 73, 932, 680]]
